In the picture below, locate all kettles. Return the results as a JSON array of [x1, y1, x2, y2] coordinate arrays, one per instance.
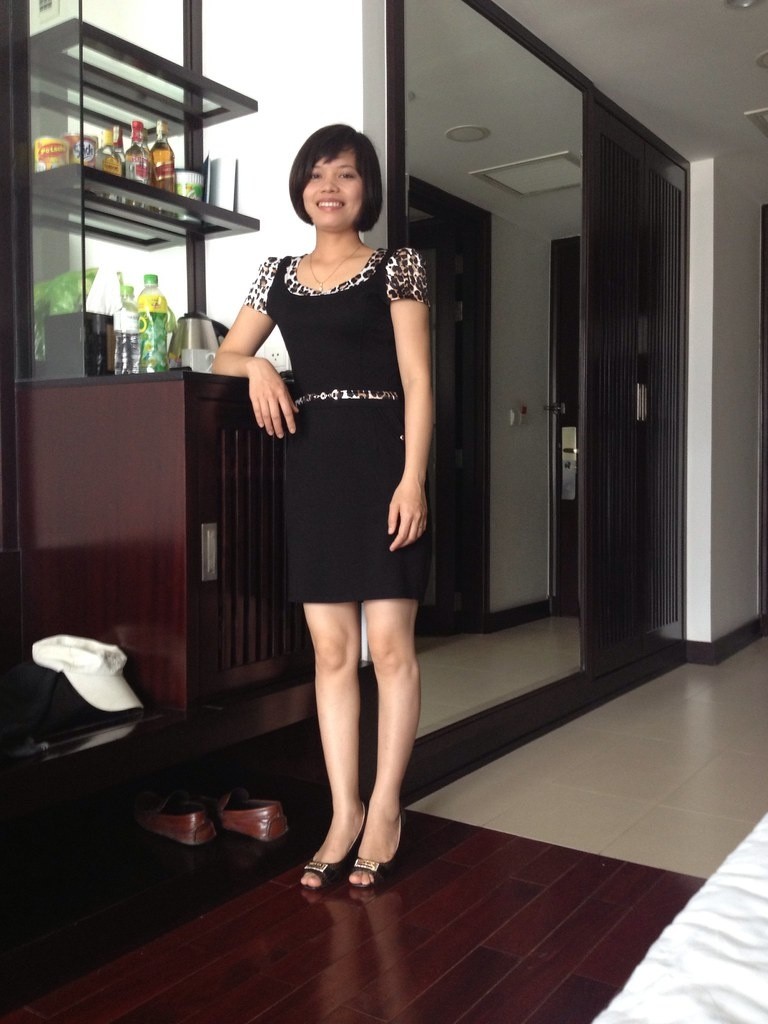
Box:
[[168, 309, 229, 367]]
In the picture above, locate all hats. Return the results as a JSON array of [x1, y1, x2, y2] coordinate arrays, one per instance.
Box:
[[32, 635, 144, 711]]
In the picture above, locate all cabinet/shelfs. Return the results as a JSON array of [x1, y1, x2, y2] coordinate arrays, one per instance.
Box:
[[14, 0, 312, 720]]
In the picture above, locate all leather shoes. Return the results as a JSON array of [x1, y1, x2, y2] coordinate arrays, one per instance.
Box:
[[196, 787, 288, 841], [133, 790, 216, 845]]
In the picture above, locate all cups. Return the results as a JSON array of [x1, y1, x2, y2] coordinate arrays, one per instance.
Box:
[[182, 349, 215, 373]]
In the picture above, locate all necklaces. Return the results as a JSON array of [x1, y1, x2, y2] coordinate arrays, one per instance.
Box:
[[310, 242, 364, 292]]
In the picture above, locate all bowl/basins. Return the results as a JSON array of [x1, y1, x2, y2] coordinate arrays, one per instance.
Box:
[[175, 169, 204, 200]]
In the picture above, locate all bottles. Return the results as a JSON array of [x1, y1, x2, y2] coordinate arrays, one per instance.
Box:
[[111, 125, 126, 176], [137, 274, 168, 372], [95, 131, 122, 176], [113, 285, 140, 375], [143, 128, 150, 153], [126, 121, 149, 184], [149, 120, 174, 192]]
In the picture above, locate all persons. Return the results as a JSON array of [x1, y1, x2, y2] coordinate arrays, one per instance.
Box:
[[212, 124, 435, 890]]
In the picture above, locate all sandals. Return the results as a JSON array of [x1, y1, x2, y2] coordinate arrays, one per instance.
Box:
[[300, 801, 406, 890]]
